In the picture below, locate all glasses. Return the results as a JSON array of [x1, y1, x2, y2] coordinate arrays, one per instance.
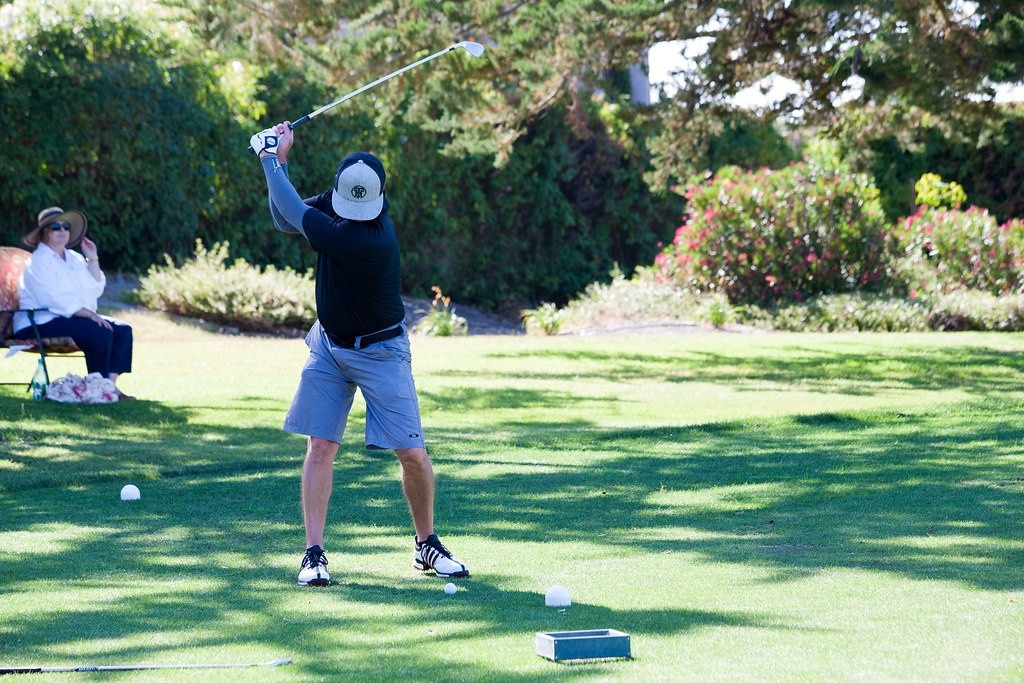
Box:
[[50, 221, 72, 231]]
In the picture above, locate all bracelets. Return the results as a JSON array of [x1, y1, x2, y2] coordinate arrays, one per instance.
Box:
[[85, 256, 98, 262]]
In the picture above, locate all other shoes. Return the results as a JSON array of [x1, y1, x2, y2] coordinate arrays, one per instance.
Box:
[[119, 392, 135, 400]]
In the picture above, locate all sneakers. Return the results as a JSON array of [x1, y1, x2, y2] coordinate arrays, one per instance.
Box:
[[298, 544, 331, 587], [413, 534, 469, 578]]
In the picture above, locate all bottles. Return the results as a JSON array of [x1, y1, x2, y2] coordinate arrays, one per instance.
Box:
[[32, 359, 47, 400]]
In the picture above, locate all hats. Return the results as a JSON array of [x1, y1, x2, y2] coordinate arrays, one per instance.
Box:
[[332, 152, 386, 220], [23, 207, 87, 248]]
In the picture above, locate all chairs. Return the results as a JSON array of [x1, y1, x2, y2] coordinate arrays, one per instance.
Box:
[[0, 246, 85, 393]]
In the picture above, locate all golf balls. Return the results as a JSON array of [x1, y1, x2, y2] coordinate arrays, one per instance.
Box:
[[444, 582, 458, 595]]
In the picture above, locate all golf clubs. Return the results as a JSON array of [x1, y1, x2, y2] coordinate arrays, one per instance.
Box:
[[0, 657, 294, 674], [247, 40, 485, 155]]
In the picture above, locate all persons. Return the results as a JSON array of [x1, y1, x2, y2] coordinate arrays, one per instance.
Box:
[[13, 206, 133, 398], [249, 121, 470, 585]]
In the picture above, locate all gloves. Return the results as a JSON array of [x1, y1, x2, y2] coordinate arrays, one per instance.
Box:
[[250, 128, 279, 156]]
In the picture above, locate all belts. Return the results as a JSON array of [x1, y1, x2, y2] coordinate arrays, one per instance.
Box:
[[326, 325, 404, 349]]
